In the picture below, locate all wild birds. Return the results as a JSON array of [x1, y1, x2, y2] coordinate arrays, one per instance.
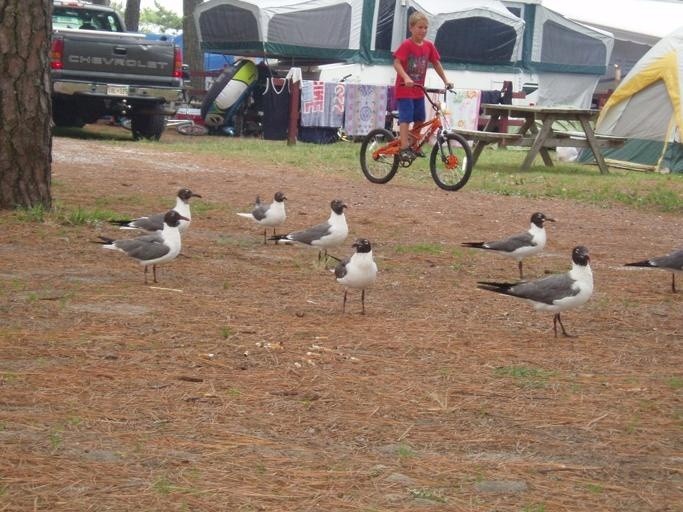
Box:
[[104, 188, 201, 236], [327, 238, 376, 314], [235, 192, 287, 245], [267, 199, 348, 271], [475, 244, 594, 338], [460, 212, 556, 279], [88, 210, 190, 284], [622, 247, 683, 293]]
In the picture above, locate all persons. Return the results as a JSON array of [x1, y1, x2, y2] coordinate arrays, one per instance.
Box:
[[390, 10, 449, 160]]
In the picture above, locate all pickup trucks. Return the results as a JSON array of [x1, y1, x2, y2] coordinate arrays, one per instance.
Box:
[[47, 5, 189, 146]]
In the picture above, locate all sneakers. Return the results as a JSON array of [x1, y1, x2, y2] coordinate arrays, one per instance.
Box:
[[399, 143, 427, 160]]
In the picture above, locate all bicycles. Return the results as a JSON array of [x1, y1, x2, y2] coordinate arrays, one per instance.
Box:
[[356, 81, 479, 192]]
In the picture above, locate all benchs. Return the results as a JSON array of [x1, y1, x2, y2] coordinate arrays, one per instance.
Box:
[[449, 129, 523, 171], [550, 128, 630, 175]]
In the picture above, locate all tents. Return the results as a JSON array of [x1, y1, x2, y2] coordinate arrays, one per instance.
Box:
[[574, 25, 682, 176]]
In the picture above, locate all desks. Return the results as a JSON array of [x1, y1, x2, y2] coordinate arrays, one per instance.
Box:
[[470, 100, 611, 173]]
